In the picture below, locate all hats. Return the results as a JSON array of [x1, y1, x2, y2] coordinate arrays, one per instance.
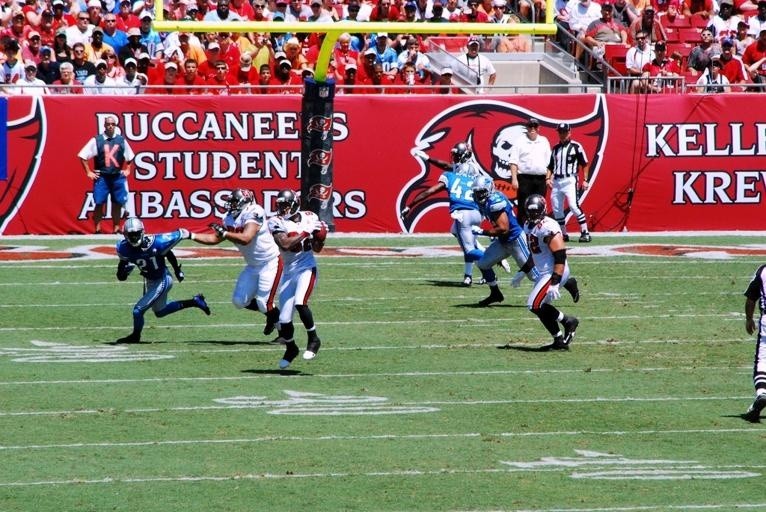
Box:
[[527, 115, 571, 132], [597, 0, 751, 68], [5, 1, 481, 78]]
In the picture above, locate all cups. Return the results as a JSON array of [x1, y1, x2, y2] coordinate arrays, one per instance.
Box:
[[409, 73, 414, 86]]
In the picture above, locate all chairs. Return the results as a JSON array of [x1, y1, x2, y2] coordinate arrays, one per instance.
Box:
[[572, 0, 766, 92]]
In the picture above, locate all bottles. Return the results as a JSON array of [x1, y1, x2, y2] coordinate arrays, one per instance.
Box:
[[619, 79, 626, 96]]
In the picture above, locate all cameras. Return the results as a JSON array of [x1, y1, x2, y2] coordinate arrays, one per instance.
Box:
[[263, 39, 268, 43]]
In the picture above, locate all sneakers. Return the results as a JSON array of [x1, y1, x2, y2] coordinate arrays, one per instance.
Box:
[[479, 294, 504, 307], [746, 394, 765, 419], [278, 340, 299, 367], [537, 315, 581, 350], [263, 309, 284, 346], [578, 231, 592, 241], [566, 277, 581, 303], [118, 335, 142, 343], [462, 276, 472, 286], [302, 333, 322, 361], [193, 291, 211, 314]]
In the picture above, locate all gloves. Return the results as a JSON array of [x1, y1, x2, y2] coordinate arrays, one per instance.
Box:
[[470, 224, 487, 236], [401, 207, 411, 220], [175, 266, 184, 281], [545, 283, 562, 303], [178, 228, 193, 242], [510, 271, 528, 290], [208, 221, 225, 238], [305, 219, 327, 242]]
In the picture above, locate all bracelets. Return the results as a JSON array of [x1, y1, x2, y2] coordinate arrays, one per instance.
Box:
[[545, 177, 550, 179]]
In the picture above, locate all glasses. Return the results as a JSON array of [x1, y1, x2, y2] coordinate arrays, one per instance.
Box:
[[105, 122, 116, 125]]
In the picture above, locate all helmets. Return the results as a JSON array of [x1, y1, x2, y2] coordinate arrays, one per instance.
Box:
[[124, 217, 147, 247], [449, 142, 494, 200], [522, 194, 548, 222], [276, 189, 302, 218], [228, 187, 255, 213]]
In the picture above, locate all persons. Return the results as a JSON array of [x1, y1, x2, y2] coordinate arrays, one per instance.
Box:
[[473, 175, 579, 306], [545, 122, 591, 243], [512, 118, 552, 229], [510, 193, 578, 349], [401, 143, 511, 285], [268, 189, 329, 369], [744, 262, 766, 419], [116, 217, 211, 344], [180, 187, 284, 335], [78, 117, 136, 234]]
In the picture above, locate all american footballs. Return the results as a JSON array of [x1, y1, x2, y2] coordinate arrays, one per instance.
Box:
[[313, 223, 326, 242]]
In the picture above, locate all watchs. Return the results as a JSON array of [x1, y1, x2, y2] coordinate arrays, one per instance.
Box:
[[583, 178, 588, 181]]
